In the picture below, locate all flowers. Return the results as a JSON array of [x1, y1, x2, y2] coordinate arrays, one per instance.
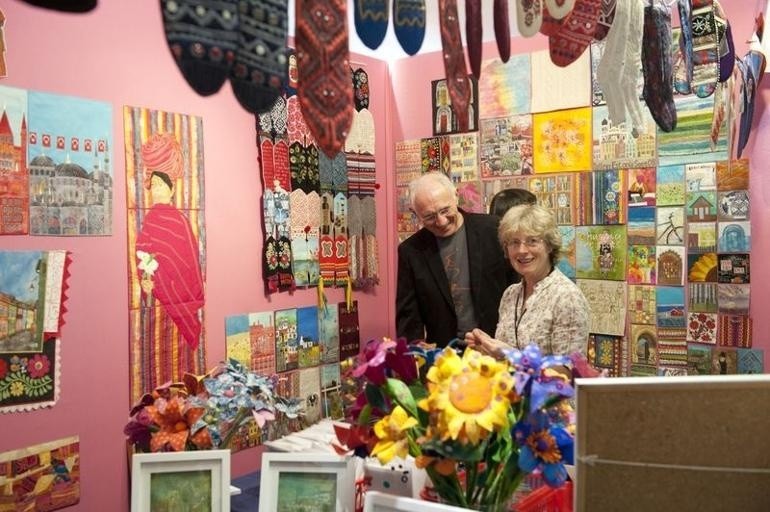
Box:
[[331, 326, 577, 511], [123, 354, 309, 452]]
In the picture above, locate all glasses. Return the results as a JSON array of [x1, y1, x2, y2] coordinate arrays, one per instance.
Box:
[[409, 199, 459, 224], [501, 236, 550, 250]]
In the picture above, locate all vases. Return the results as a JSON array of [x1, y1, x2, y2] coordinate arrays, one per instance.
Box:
[[436, 483, 515, 511]]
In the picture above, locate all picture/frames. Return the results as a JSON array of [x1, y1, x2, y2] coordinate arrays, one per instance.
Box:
[[130, 448, 233, 511], [258, 451, 358, 511], [574, 375, 770, 511], [362, 488, 473, 511]]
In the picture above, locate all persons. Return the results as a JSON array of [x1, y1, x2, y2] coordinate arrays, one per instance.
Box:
[[488, 187, 538, 218], [392, 169, 522, 357], [128, 171, 205, 399], [461, 201, 592, 366]]
